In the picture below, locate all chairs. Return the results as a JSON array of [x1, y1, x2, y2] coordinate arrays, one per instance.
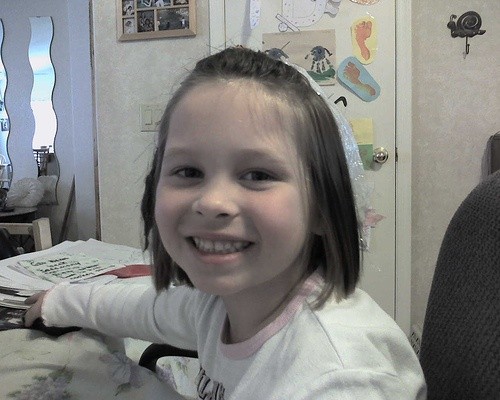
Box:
[[137, 167, 500, 400], [0, 218, 52, 251]]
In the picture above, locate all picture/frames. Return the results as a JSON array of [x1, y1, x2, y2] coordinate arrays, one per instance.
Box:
[[116, 0, 197, 42]]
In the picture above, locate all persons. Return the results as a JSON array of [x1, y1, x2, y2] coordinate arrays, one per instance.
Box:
[[23, 45, 427, 400]]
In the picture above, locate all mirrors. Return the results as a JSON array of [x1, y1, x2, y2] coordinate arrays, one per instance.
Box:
[[1, 17, 59, 207]]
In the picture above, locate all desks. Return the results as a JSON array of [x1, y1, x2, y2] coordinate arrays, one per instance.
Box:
[[0, 208, 37, 259]]
